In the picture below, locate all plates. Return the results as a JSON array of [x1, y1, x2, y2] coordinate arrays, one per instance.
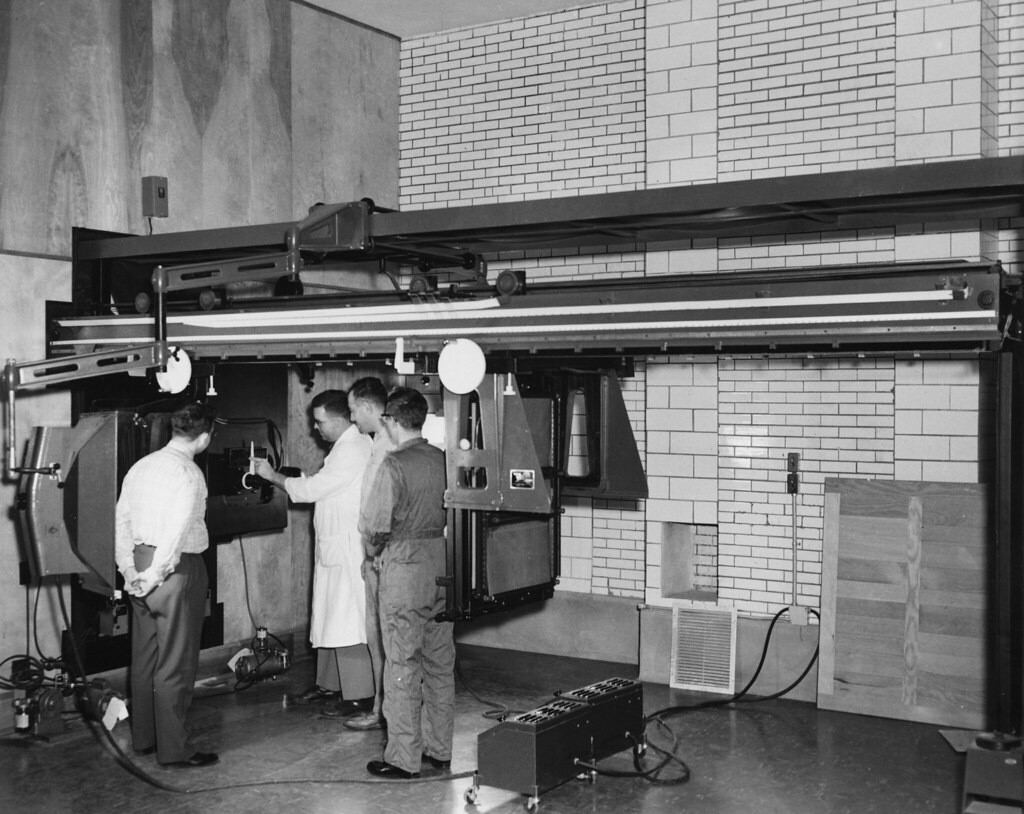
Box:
[[155, 347, 192, 394], [438, 339, 486, 394]]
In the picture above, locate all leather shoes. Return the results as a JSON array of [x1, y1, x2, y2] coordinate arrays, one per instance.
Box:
[[366, 760, 420, 779], [133, 743, 157, 756], [421, 752, 451, 770], [319, 699, 369, 717], [158, 751, 219, 768], [300, 685, 341, 703]]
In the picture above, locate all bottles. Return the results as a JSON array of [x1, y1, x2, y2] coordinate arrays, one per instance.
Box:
[[14, 705, 29, 730]]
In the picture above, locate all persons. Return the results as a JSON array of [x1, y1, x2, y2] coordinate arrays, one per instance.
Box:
[[250, 390, 375, 719], [343, 375, 398, 729], [358, 386, 456, 779], [114, 404, 218, 770]]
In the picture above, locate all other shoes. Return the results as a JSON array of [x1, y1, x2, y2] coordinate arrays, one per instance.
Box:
[[342, 712, 387, 730]]
